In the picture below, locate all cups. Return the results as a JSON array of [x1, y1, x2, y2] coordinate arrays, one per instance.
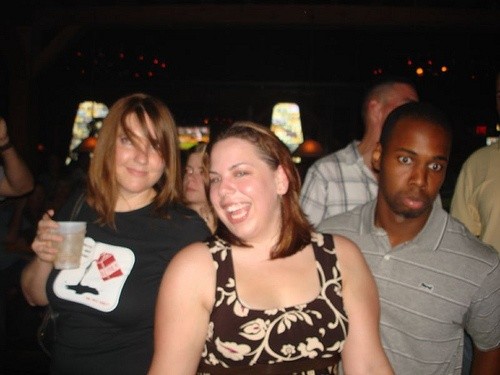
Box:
[[53, 221, 87, 270]]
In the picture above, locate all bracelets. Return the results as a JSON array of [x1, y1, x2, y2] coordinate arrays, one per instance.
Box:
[[0, 140, 14, 151]]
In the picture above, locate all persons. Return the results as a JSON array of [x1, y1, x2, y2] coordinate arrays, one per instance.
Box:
[[148, 121, 397, 374], [0, 92, 222, 374], [297, 75, 500, 375]]
